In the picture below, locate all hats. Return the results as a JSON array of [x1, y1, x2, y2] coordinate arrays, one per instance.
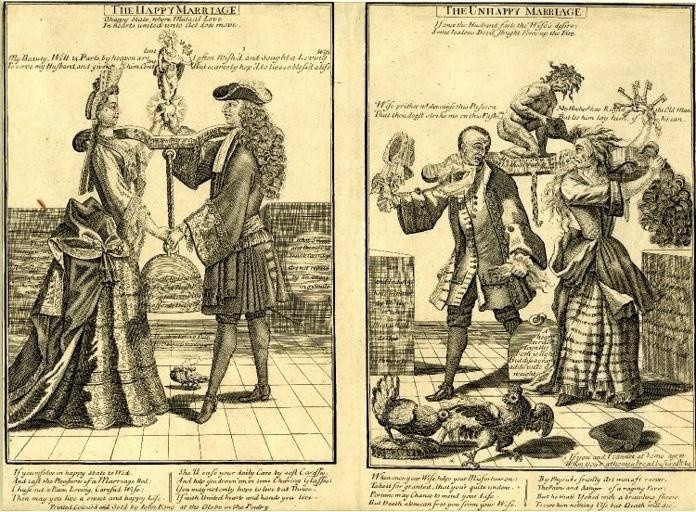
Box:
[[213, 81, 274, 104]]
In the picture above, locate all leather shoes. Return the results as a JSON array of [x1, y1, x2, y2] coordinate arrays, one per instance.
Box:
[[194, 392, 219, 424], [237, 384, 272, 402], [426, 384, 457, 404]]
